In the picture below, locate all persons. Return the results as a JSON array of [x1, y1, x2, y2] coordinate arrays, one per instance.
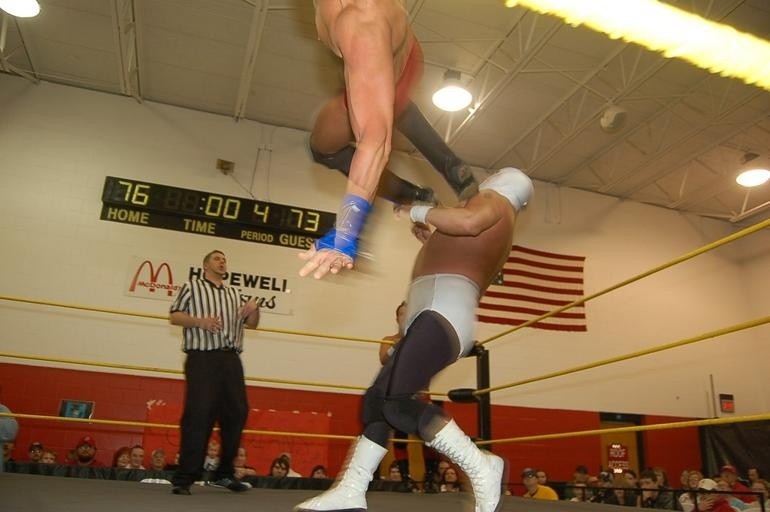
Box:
[[298, 2, 479, 278], [169, 249, 259, 495], [0, 402, 19, 462], [31, 434, 104, 468], [522, 462, 769, 511], [374, 458, 462, 492], [290, 167, 534, 511], [205, 440, 328, 479], [112, 444, 181, 471]]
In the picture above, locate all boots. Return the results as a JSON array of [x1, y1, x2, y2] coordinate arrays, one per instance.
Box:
[[425, 417, 504, 512], [296, 434, 389, 511]]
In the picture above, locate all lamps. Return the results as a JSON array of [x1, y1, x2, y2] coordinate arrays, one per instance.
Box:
[[431, 69, 473, 115], [735, 150, 769, 190]]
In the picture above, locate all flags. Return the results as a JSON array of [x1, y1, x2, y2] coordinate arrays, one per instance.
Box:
[[472, 245, 587, 331]]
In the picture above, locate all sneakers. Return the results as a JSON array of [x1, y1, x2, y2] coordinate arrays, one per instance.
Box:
[[448, 162, 477, 201], [418, 186, 437, 204], [208, 473, 253, 492]]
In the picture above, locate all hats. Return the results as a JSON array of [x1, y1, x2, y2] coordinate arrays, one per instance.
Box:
[[698, 478, 718, 490], [720, 465, 738, 474], [77, 435, 96, 450]]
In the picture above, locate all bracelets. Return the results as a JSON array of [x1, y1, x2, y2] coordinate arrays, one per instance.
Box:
[[408, 205, 434, 223]]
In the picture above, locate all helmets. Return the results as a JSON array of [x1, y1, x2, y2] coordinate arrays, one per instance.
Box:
[[478, 167, 534, 212]]
